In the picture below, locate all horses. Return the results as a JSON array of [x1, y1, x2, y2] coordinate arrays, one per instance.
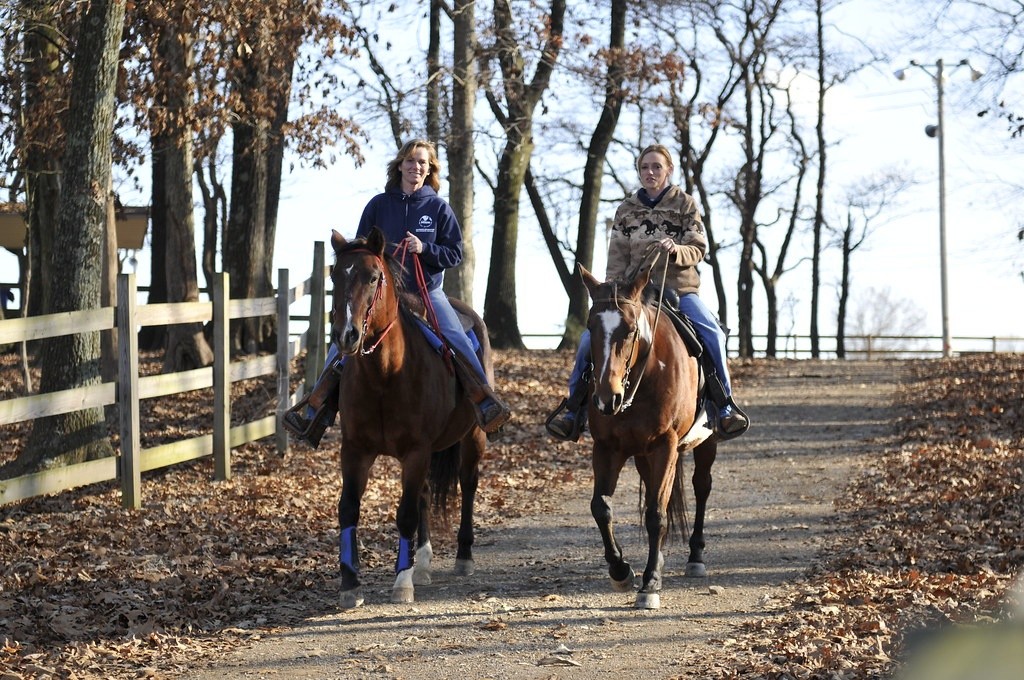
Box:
[[331, 225, 494, 609], [576, 261, 723, 610]]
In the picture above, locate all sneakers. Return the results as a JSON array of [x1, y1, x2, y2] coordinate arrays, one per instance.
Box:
[[484, 406, 504, 442], [549, 418, 586, 442], [712, 414, 747, 444], [286, 411, 328, 451]]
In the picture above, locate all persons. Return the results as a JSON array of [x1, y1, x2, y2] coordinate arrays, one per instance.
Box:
[[284, 138, 505, 449], [549, 145, 747, 444]]
